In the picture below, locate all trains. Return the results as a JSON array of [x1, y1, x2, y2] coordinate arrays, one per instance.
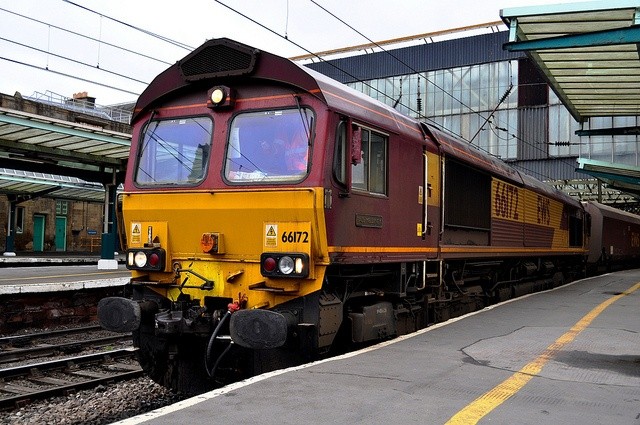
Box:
[[95, 35, 639, 393]]
